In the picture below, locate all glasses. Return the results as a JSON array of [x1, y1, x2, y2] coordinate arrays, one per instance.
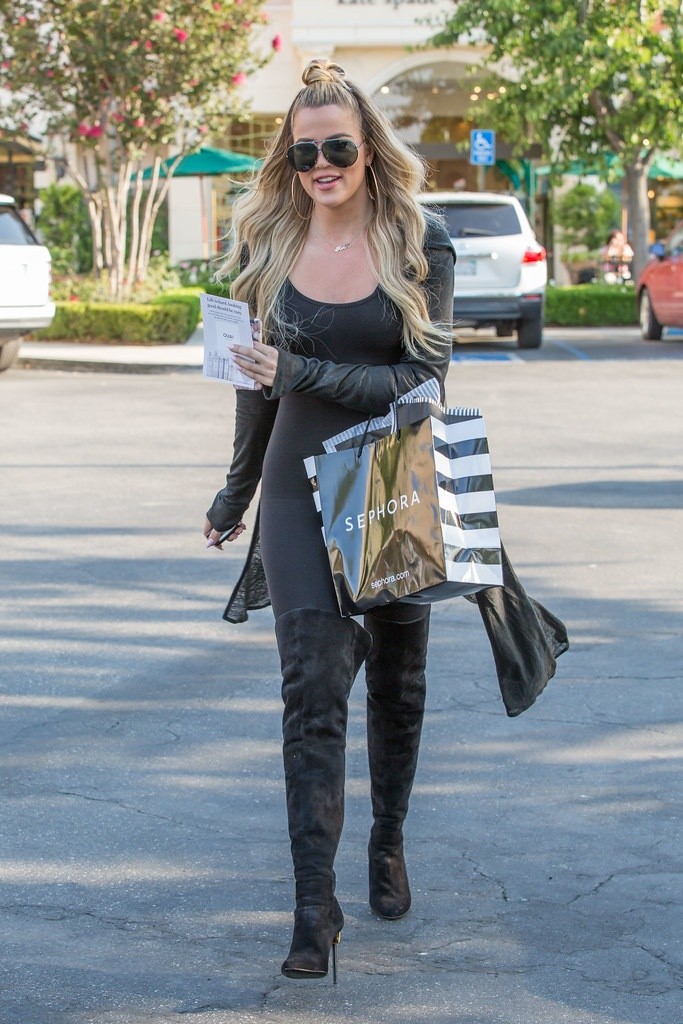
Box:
[[285, 139, 366, 173]]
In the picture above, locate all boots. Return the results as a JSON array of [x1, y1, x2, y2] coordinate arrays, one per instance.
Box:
[[363, 605, 431, 919], [274, 607, 373, 982]]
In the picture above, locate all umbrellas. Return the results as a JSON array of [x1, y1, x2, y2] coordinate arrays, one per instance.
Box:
[[534, 141, 683, 234], [130, 142, 267, 260]]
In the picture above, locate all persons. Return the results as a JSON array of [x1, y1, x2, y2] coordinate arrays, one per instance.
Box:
[[203, 61, 455, 979], [598, 225, 683, 285]]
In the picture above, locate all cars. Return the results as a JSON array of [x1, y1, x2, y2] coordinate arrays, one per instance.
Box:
[[634, 219, 683, 340]]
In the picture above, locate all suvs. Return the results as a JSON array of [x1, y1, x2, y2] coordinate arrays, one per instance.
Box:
[[0, 191, 57, 373], [415, 193, 548, 350]]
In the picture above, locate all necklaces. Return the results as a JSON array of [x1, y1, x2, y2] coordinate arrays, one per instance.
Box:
[[314, 225, 365, 254]]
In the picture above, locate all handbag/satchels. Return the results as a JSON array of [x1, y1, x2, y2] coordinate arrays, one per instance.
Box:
[[302, 361, 504, 617]]
